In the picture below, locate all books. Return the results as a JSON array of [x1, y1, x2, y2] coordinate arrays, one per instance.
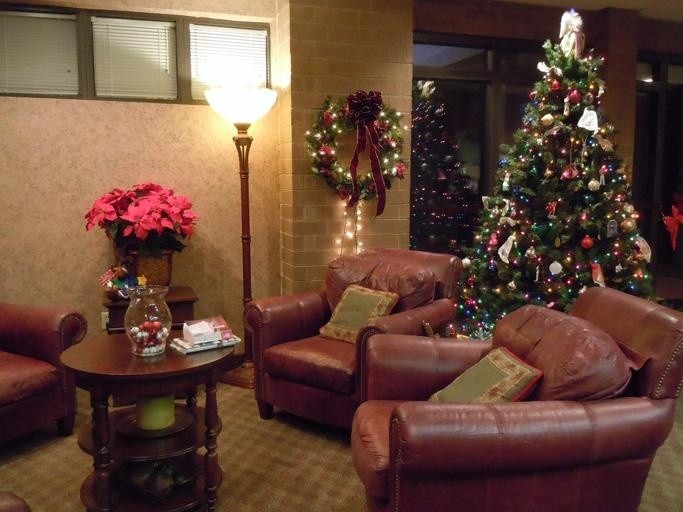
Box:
[[169, 315, 242, 355]]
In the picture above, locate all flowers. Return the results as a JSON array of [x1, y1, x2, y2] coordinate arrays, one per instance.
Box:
[[79, 179, 201, 258], [302, 92, 409, 206]]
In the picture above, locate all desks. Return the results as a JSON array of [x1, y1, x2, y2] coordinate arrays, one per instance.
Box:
[[58, 325, 249, 510]]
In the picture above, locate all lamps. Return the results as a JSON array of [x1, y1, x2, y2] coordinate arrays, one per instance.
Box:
[[203, 81, 280, 392]]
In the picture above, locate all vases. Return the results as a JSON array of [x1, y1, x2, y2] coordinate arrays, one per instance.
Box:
[[115, 244, 175, 291]]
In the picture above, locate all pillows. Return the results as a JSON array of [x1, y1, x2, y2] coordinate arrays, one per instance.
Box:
[[317, 280, 400, 344], [427, 340, 546, 408]]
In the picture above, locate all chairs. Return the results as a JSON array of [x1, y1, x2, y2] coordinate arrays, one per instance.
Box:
[[344, 281, 681, 511], [1, 299, 90, 466], [243, 240, 465, 434]]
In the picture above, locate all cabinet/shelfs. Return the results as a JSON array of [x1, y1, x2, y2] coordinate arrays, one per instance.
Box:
[[101, 284, 198, 337]]
